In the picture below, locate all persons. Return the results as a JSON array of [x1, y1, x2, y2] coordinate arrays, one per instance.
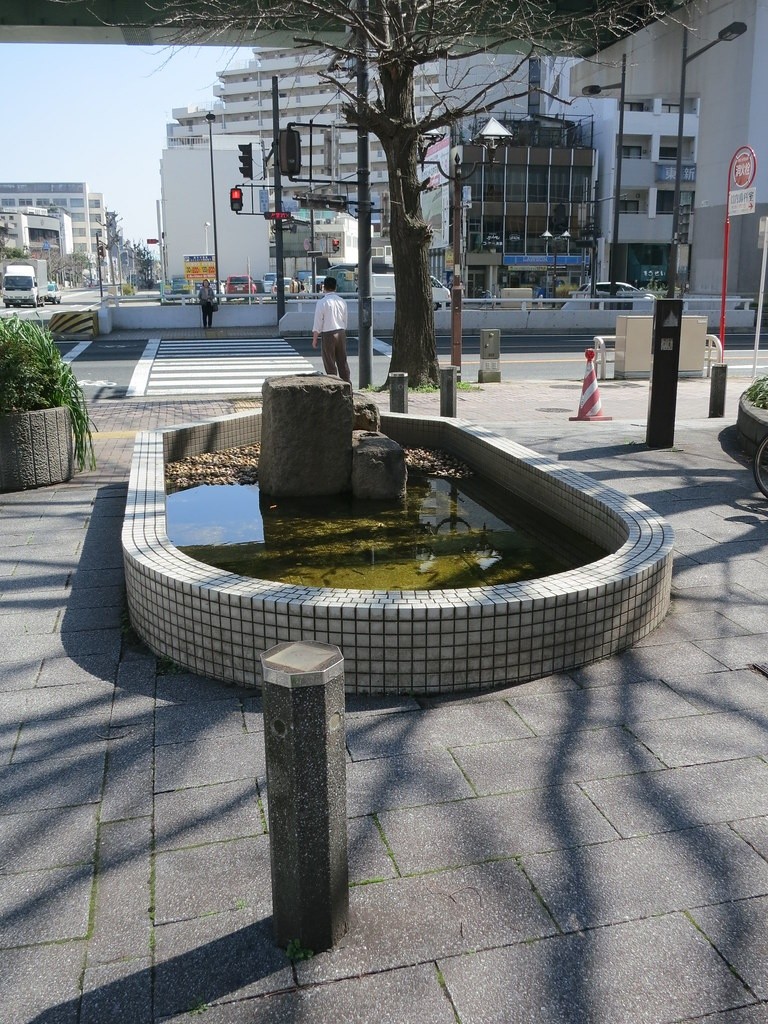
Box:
[[198, 279, 214, 328], [147, 278, 152, 290], [6, 279, 15, 287], [271, 276, 324, 300], [476, 285, 483, 297], [447, 279, 453, 291], [537, 287, 544, 307], [312, 276, 350, 383]]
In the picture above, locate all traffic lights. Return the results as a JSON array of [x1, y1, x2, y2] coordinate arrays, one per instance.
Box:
[[238, 142, 253, 181], [230, 188, 243, 212], [332, 239, 339, 252]]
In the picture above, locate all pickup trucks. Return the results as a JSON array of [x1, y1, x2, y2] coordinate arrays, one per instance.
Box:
[[225, 276, 257, 301]]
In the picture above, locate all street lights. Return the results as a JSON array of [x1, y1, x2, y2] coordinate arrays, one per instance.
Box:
[[539, 230, 571, 309], [581, 52, 626, 309], [668, 20, 749, 298], [203, 222, 210, 255], [204, 111, 219, 305], [414, 116, 514, 381]]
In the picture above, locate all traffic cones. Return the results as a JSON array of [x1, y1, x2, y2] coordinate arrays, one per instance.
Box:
[[567, 348, 614, 422]]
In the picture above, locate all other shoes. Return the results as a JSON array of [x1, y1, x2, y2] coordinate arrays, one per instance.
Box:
[[208, 325, 211, 329], [204, 322, 207, 328]]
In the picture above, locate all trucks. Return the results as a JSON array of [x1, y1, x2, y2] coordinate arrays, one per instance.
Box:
[[2, 258, 48, 308]]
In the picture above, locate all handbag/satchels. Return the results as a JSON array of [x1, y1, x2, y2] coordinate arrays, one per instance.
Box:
[[208, 300, 218, 312]]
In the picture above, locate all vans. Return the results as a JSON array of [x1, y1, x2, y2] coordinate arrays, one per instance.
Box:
[[372, 274, 451, 311]]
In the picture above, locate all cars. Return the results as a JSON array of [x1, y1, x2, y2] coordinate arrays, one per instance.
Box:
[[47, 283, 61, 305], [162, 276, 227, 303], [262, 270, 327, 299], [574, 282, 656, 310]]
